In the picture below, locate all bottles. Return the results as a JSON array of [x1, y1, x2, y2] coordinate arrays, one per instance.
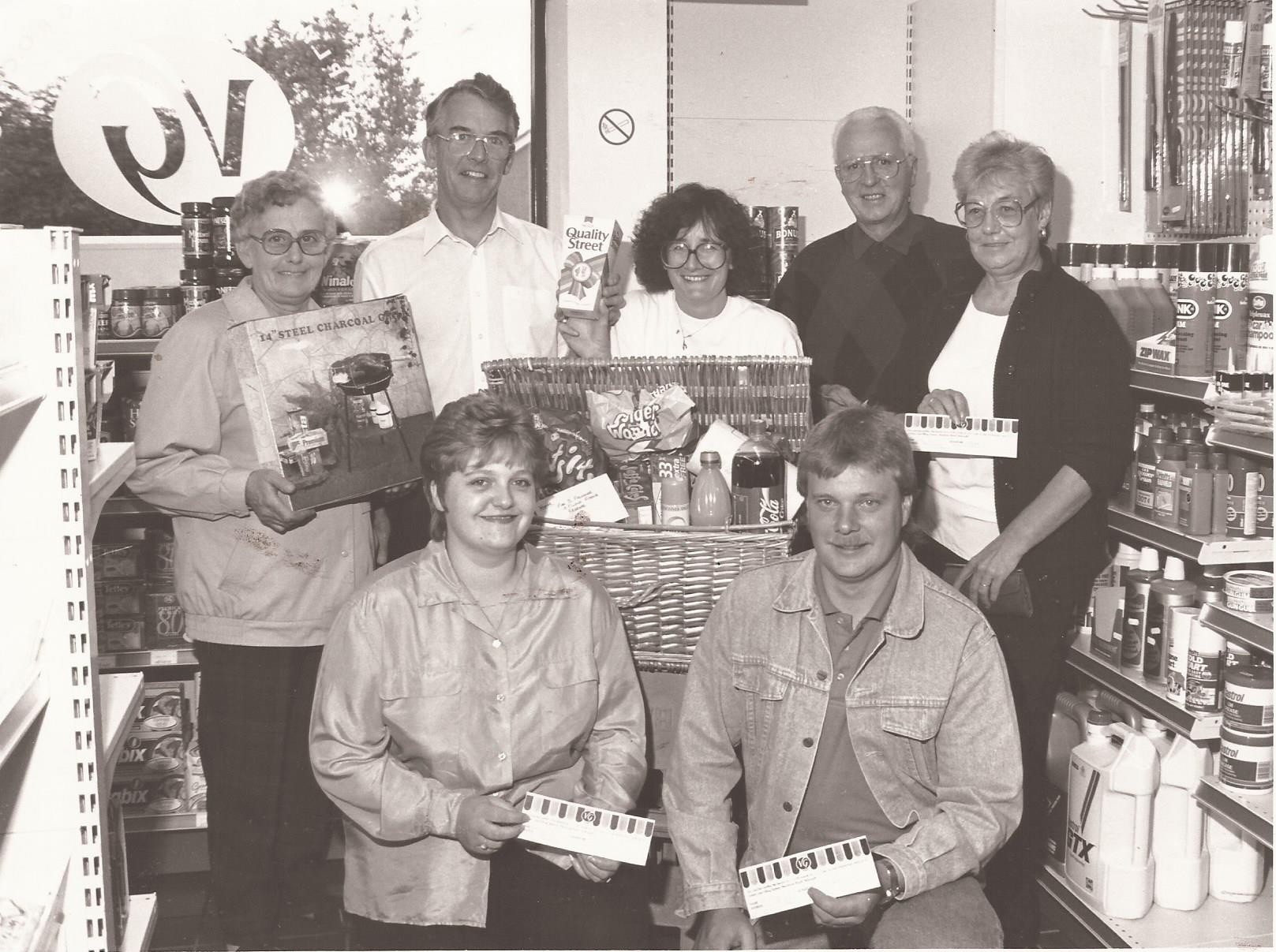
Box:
[[731, 419, 786, 534], [1044, 239, 1274, 920], [661, 476, 689, 526], [689, 451, 731, 526], [109, 196, 247, 338], [353, 398, 368, 430]]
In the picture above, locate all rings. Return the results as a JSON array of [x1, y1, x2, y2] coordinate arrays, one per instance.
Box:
[[562, 316, 568, 323], [927, 398, 933, 404], [480, 838, 487, 849], [606, 877, 613, 884], [980, 583, 991, 586]]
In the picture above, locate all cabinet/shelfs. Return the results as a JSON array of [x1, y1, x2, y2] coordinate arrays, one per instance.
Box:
[[0, 229, 387, 952], [1039, 369, 1273, 949]]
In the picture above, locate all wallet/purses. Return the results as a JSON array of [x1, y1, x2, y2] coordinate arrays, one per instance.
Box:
[[942, 562, 1035, 619]]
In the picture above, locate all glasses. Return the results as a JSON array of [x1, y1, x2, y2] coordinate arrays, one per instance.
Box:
[[248, 229, 329, 256], [431, 131, 511, 160], [660, 240, 729, 270], [955, 192, 1042, 228], [838, 153, 911, 183]]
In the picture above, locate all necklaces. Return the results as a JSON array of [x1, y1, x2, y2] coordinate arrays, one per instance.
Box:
[[676, 303, 722, 350]]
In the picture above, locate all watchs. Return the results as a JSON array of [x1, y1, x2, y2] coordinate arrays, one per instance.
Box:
[[876, 860, 903, 911]]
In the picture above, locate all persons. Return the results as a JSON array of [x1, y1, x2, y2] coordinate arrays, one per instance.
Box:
[[891, 131, 1131, 948], [661, 406, 1023, 950], [352, 72, 623, 569], [308, 395, 647, 951], [555, 182, 805, 386], [765, 108, 981, 555], [124, 172, 419, 952]]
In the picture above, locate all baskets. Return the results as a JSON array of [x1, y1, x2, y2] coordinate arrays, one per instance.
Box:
[[478, 350, 816, 672]]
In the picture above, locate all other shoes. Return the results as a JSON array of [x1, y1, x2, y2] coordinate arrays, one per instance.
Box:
[[221, 904, 309, 940], [269, 900, 330, 933]]
